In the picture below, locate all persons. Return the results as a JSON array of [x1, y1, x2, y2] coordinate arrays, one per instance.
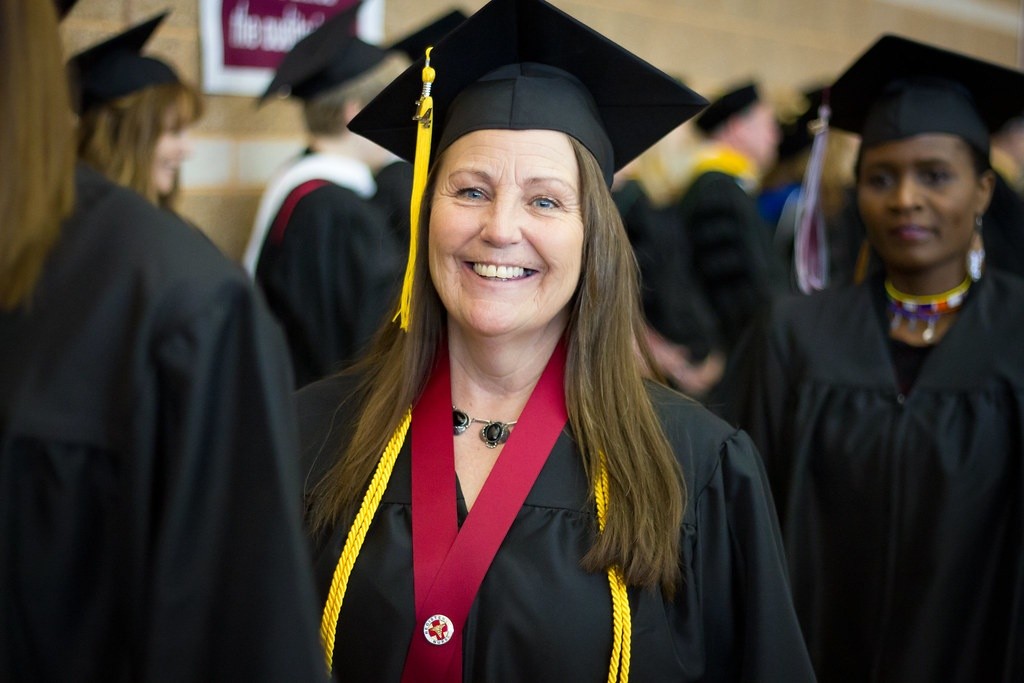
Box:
[[244, 0, 470, 390], [721, 33, 1024, 683], [1, 0, 331, 682], [66, 9, 203, 217], [611, 81, 866, 411], [297, 0, 812, 682], [981, 122, 1024, 278]]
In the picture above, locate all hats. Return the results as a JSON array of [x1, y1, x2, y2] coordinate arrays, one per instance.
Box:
[[386, 9, 465, 63], [346, 0, 710, 195], [66, 11, 178, 117], [815, 34, 1024, 172], [256, 0, 384, 109], [776, 84, 836, 156], [695, 84, 759, 135]]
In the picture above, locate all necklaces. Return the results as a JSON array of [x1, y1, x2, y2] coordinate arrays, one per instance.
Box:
[[452, 405, 517, 448], [884, 276, 971, 340]]
